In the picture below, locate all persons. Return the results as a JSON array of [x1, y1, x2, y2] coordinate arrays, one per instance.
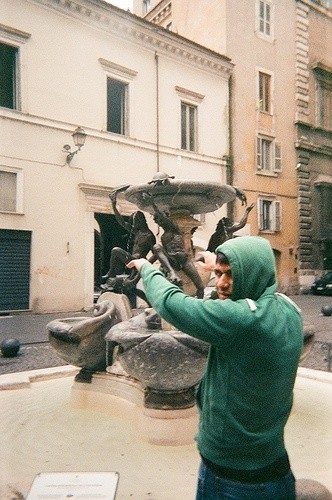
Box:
[[141, 191, 204, 299], [96, 191, 156, 308], [206, 203, 256, 254], [125, 236, 306, 500]]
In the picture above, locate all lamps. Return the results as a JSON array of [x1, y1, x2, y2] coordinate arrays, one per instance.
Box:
[[62, 126, 88, 164]]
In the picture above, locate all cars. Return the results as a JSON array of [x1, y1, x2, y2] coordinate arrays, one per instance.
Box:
[[309, 270, 332, 296]]
